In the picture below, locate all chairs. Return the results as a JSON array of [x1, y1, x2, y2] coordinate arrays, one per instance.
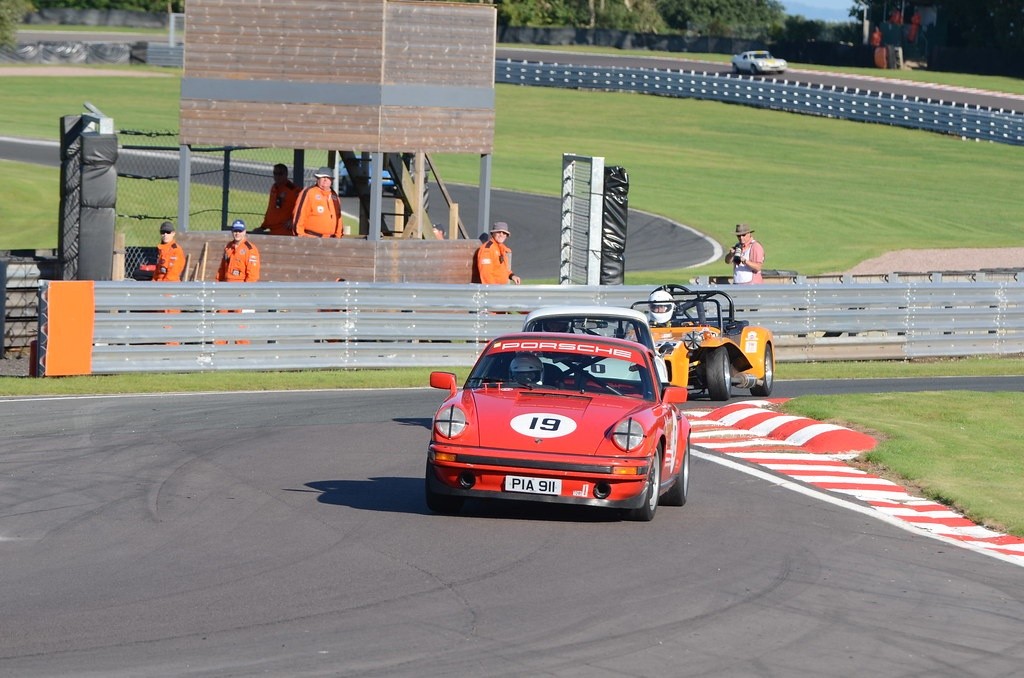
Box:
[[544, 363, 562, 387]]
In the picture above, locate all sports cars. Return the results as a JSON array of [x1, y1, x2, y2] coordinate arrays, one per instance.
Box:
[[623, 283, 775, 402]]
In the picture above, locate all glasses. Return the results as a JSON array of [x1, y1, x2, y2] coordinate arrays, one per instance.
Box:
[[160, 230, 171, 234], [737, 234, 746, 237], [234, 229, 244, 233]]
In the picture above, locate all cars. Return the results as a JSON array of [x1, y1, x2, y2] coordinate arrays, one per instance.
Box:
[[425, 304, 705, 522], [329, 156, 398, 198], [730, 50, 789, 76]]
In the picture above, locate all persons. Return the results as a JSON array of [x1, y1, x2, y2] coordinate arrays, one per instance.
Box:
[[433, 224, 444, 240], [510, 354, 544, 385], [649, 290, 674, 327], [477, 222, 520, 343], [292, 168, 344, 238], [152, 222, 186, 345], [252, 164, 302, 236], [725, 224, 765, 284], [213, 219, 260, 345]]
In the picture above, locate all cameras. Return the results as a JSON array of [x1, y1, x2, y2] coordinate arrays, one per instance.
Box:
[[732, 244, 743, 264]]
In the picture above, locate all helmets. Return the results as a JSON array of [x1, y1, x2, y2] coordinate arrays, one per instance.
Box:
[[509, 353, 544, 386], [648, 291, 675, 323], [541, 320, 572, 334]]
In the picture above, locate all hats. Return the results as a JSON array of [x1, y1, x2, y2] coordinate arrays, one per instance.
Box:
[[431, 223, 445, 234], [313, 166, 335, 180], [231, 220, 246, 232], [732, 224, 755, 235], [159, 222, 175, 232], [489, 222, 510, 237]]
